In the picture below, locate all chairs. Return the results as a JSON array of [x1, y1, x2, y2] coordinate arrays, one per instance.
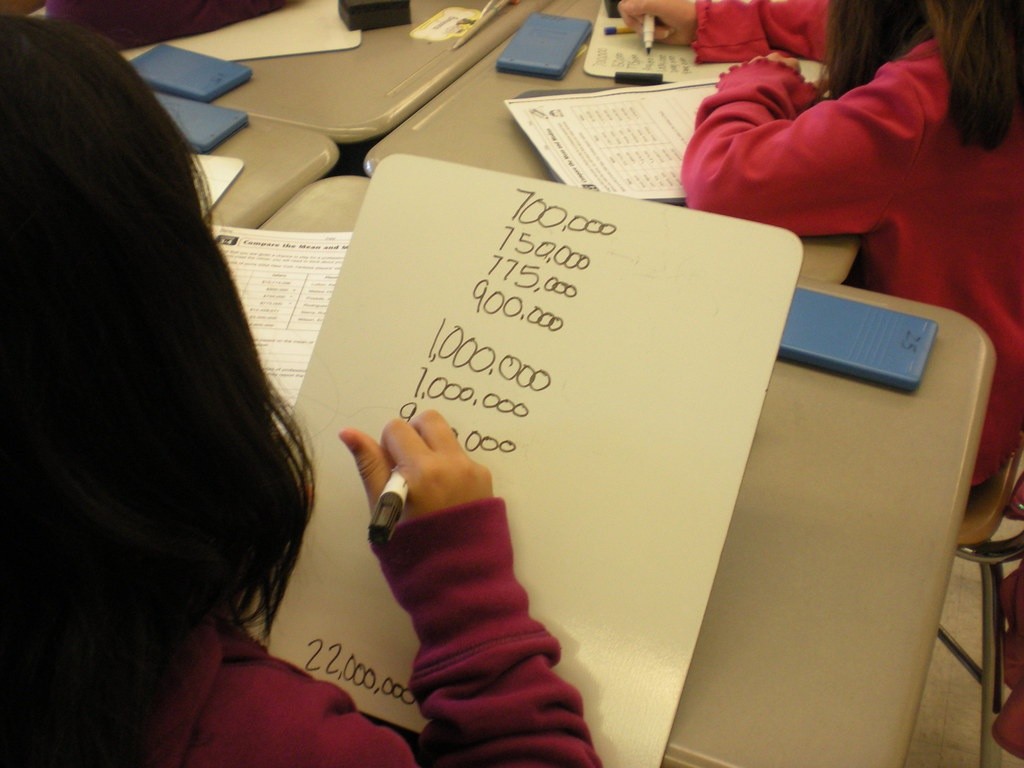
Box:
[[939, 427, 1024, 768]]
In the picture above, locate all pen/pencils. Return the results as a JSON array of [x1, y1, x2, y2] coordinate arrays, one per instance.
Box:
[[604, 26, 637, 34]]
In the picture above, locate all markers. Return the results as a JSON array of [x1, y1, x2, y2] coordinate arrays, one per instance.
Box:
[[643, 13, 656, 55], [367, 466, 410, 545]]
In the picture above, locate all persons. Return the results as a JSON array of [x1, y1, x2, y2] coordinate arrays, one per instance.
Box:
[[0, 0, 601, 766], [620, 0, 1024, 492]]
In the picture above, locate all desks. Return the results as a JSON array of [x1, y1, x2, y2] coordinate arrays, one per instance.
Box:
[[28, 0, 994, 768]]
